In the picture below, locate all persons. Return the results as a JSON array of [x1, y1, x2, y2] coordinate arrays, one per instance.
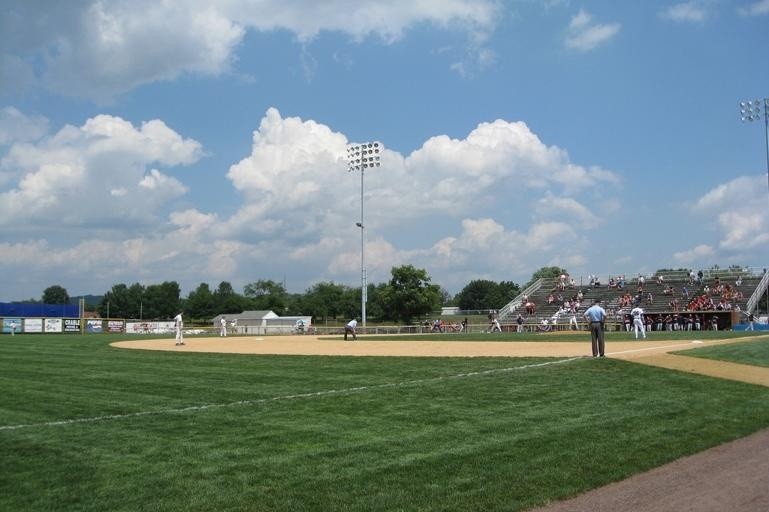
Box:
[[582, 297, 606, 359], [628, 303, 648, 340], [343, 316, 360, 341], [483, 270, 755, 332], [9, 320, 18, 336], [173, 309, 184, 346], [230, 320, 238, 334], [219, 316, 227, 336], [419, 316, 470, 335], [298, 322, 304, 332]]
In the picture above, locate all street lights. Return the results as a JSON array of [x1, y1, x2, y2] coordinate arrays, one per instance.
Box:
[[739, 95, 768, 191], [345, 141, 384, 335]]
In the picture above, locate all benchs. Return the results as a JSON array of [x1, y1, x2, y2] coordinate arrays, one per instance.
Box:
[[497, 277, 763, 332]]
